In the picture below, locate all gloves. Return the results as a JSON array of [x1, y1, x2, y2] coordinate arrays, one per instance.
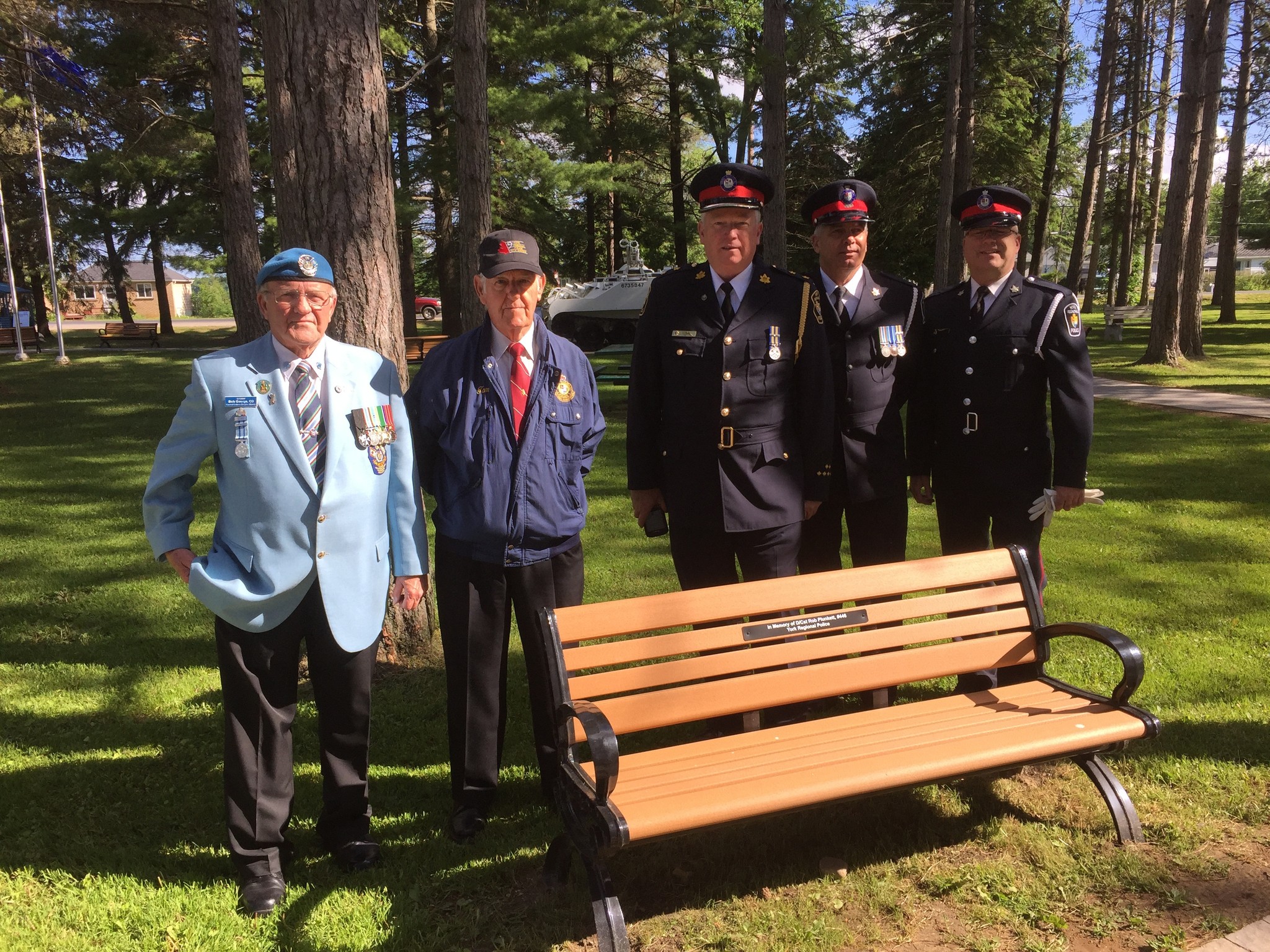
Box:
[[1044, 488, 1104, 512], [1028, 495, 1053, 527]]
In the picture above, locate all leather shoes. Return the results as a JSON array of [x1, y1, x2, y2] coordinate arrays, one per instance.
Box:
[[323, 833, 383, 873], [239, 870, 285, 917], [450, 812, 488, 844]]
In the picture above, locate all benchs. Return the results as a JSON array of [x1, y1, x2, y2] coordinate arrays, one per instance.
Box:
[[403, 335, 452, 364], [0, 326, 42, 355], [1103, 304, 1154, 341], [98, 323, 160, 348], [533, 543, 1166, 952], [592, 364, 631, 386]]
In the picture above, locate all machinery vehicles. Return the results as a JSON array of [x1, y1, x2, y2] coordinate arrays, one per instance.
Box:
[[545, 239, 675, 351]]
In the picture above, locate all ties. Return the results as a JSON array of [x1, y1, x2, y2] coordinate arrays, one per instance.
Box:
[[833, 286, 852, 331], [294, 361, 327, 495], [720, 282, 736, 331], [971, 286, 991, 321], [507, 342, 532, 442]]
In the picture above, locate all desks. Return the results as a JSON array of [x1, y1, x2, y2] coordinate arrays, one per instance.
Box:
[[594, 344, 634, 356]]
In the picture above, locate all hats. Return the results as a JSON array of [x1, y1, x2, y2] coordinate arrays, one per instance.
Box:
[[480, 230, 544, 279], [801, 179, 876, 227], [690, 162, 774, 213], [256, 248, 335, 291], [951, 185, 1032, 231]]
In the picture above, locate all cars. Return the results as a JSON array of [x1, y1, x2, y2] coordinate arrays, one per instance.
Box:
[[415, 295, 442, 319]]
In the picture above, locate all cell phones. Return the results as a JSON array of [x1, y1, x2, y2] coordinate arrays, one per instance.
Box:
[[643, 505, 669, 538]]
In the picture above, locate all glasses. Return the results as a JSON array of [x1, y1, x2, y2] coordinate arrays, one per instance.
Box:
[[264, 289, 335, 306]]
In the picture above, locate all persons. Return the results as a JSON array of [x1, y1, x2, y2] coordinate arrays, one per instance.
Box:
[[796, 177, 938, 710], [403, 226, 607, 843], [628, 162, 839, 741], [904, 184, 1093, 696], [141, 244, 430, 917]]
